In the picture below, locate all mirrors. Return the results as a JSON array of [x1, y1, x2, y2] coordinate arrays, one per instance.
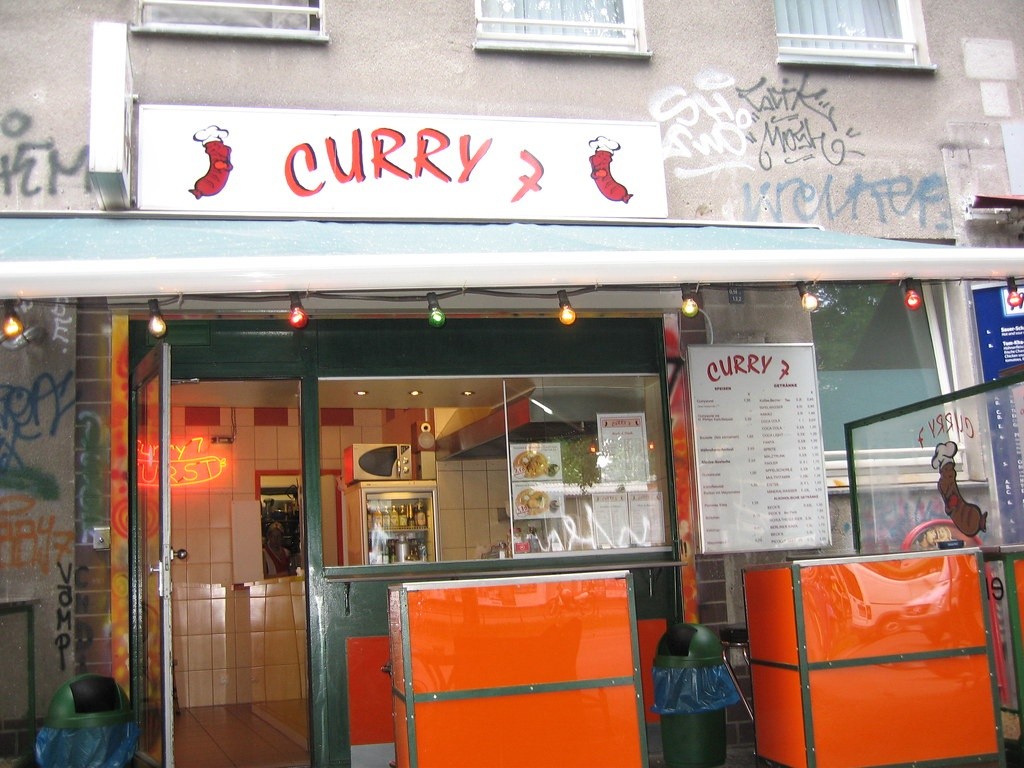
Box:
[[254, 468, 306, 583]]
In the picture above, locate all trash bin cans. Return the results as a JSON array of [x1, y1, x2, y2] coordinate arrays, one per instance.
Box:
[[650, 620, 735, 768], [34, 673, 141, 768]]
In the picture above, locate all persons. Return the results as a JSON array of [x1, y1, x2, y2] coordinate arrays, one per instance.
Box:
[[263, 523, 294, 578]]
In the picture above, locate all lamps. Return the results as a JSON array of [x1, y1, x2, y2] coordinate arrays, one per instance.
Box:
[[1007, 277, 1023, 307], [557, 290, 576, 325], [146, 299, 167, 338], [797, 281, 818, 312], [288, 292, 308, 329], [681, 284, 698, 317], [905, 278, 922, 311], [426, 292, 446, 327], [2, 299, 24, 337]]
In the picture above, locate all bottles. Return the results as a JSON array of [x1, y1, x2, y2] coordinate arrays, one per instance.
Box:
[[416, 501, 426, 527], [398, 504, 407, 529], [390, 505, 400, 528], [372, 506, 382, 526], [407, 503, 416, 529], [366, 502, 373, 528], [381, 506, 390, 526], [366, 533, 428, 565], [498, 527, 540, 558]]
